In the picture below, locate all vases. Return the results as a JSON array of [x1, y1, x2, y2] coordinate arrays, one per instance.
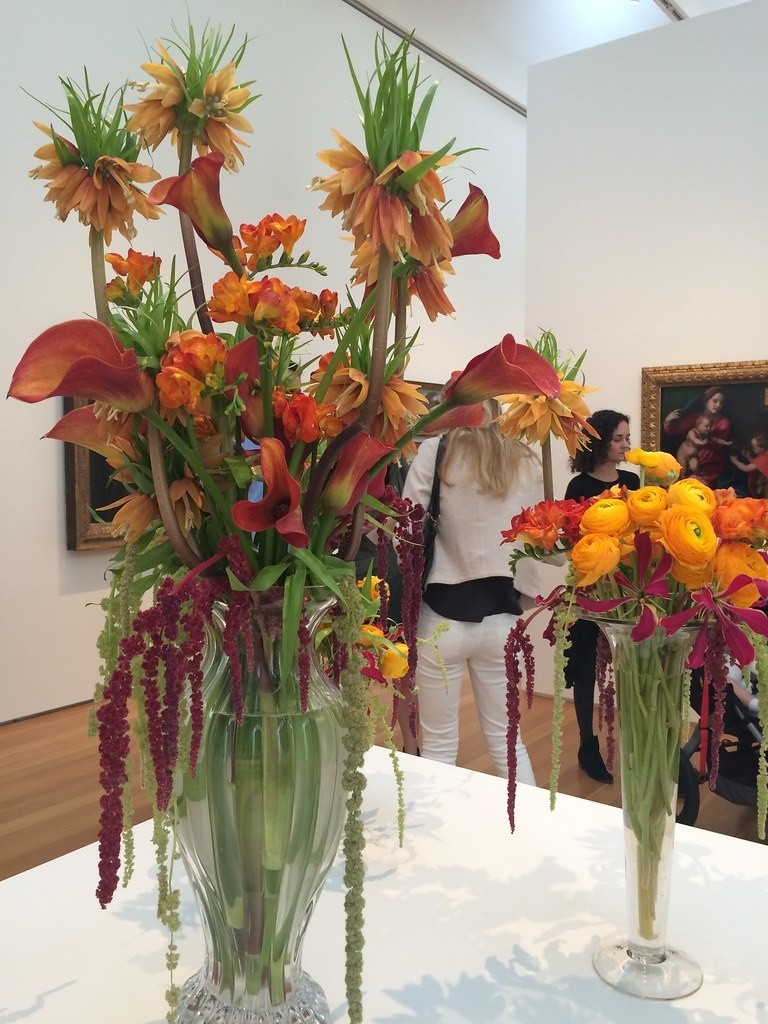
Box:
[[165, 582, 351, 1023], [578, 612, 714, 1003]]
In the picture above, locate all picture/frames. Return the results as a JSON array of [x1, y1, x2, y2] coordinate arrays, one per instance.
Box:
[[66, 384, 126, 553], [640, 358, 768, 501]]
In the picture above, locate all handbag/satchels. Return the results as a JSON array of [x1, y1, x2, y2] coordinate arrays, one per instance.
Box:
[[411, 515, 437, 590]]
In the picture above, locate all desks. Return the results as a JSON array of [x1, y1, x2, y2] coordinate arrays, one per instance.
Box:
[[0, 743, 768, 1023]]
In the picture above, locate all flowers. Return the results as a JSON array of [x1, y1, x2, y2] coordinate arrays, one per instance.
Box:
[[4, 16, 601, 985], [484, 445, 768, 834]]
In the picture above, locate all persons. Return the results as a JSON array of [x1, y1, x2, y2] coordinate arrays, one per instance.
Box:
[[391, 369, 544, 788], [724, 654, 759, 718], [562, 409, 645, 784], [228, 359, 422, 758]]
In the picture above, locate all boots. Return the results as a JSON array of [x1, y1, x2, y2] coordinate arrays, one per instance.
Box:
[[578, 735, 615, 784]]
